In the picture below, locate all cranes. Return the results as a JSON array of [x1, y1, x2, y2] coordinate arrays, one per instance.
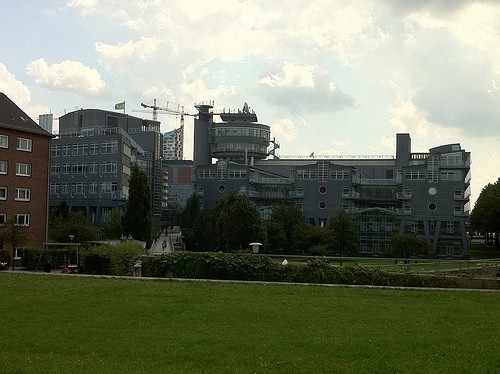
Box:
[[136, 98, 257, 162]]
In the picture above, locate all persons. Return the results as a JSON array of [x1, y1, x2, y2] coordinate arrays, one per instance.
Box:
[[60, 255, 70, 273], [154, 231, 179, 250], [44, 259, 52, 273]]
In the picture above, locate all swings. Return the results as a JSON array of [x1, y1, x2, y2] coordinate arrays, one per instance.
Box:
[[44, 246, 52, 274], [63, 245, 72, 274]]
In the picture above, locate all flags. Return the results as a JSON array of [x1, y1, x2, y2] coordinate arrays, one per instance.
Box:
[[115, 101, 125, 109]]
